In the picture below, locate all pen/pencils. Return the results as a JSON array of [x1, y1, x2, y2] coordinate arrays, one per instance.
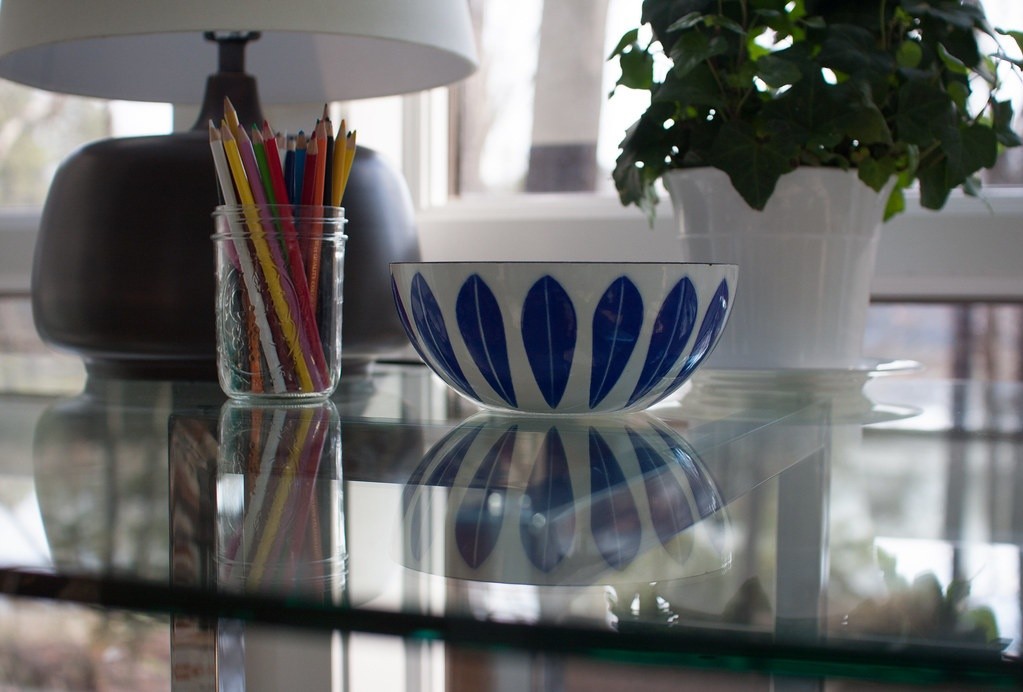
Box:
[[207, 93, 357, 398]]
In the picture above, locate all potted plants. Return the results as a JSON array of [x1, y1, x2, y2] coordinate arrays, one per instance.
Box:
[[607, 391, 1008, 657], [608, 0, 1023, 380]]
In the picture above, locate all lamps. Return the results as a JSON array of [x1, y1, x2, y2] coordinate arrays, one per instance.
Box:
[[0, 1, 481, 387], [32, 376, 253, 650]]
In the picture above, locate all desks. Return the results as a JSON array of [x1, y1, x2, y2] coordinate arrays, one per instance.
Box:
[[1, 346, 935, 664]]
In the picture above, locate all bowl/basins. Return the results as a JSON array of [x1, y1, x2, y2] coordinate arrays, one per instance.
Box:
[[389, 257, 739, 418], [389, 400, 734, 584]]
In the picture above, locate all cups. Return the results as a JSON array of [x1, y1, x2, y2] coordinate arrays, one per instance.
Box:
[[213, 203, 348, 400], [216, 393, 347, 592]]
